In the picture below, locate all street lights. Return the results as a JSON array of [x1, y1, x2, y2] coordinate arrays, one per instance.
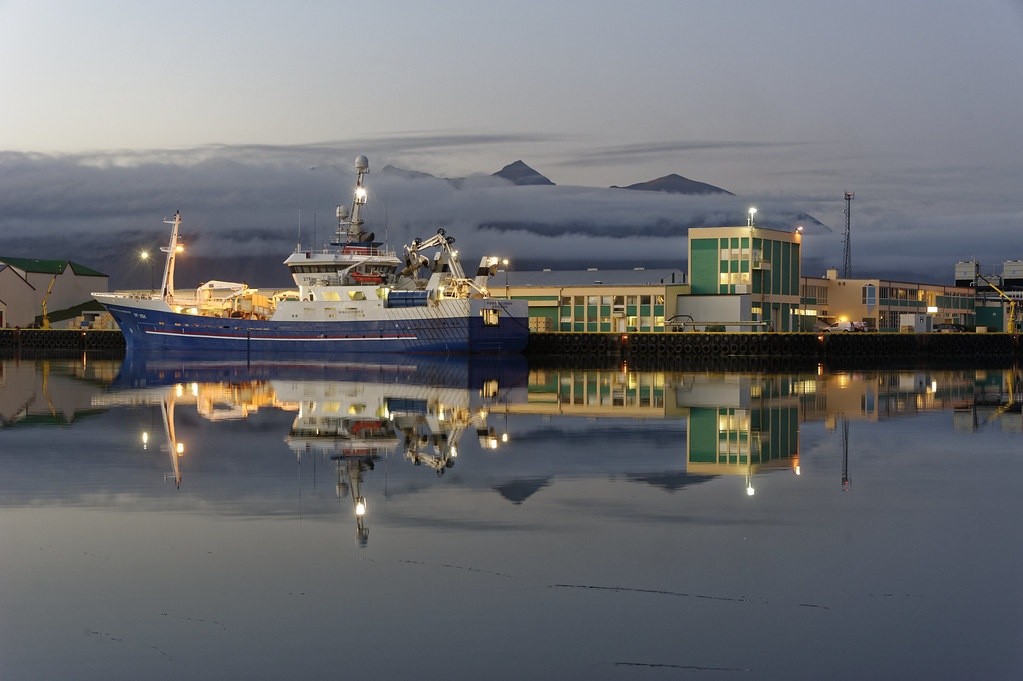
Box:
[[748, 207, 758, 227], [142, 404, 155, 451], [502, 258, 510, 300], [140, 251, 155, 290], [173, 245, 184, 290], [500, 413, 510, 443]]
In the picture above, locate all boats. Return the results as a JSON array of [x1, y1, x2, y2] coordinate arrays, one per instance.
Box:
[[349, 271, 389, 286], [91, 349, 531, 547], [351, 421, 382, 436], [90, 152, 531, 354]]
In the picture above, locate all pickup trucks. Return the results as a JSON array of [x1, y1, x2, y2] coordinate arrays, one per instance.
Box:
[[822, 321, 855, 333]]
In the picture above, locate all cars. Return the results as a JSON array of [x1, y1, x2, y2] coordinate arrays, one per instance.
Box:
[[933, 322, 974, 332]]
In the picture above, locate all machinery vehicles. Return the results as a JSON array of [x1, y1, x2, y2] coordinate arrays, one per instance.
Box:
[[40, 359, 56, 419], [976, 272, 1021, 334], [36, 265, 63, 329], [980, 369, 1023, 425]]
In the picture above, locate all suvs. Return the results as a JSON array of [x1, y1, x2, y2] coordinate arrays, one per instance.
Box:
[[853, 321, 875, 332]]
